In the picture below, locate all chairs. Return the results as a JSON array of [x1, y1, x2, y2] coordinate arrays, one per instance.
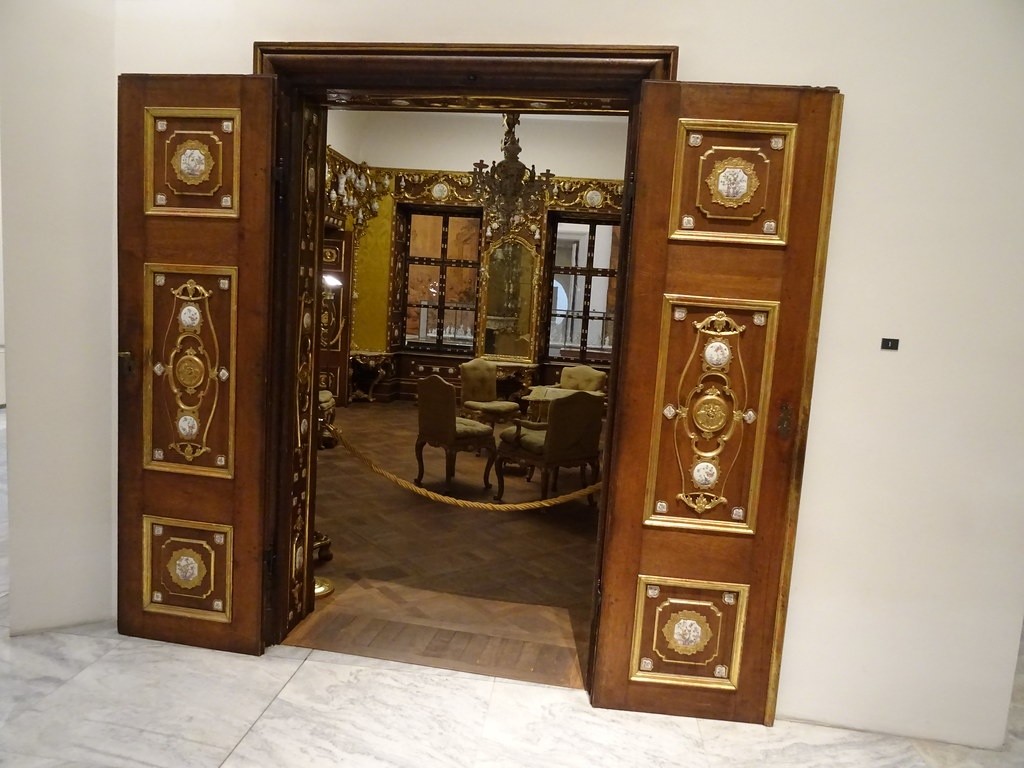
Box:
[[413, 375, 497, 498], [493, 390, 605, 511], [460, 357, 521, 457], [525, 365, 607, 390]]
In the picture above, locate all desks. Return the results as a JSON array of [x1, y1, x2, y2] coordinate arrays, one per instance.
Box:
[[523, 393, 605, 483], [350, 350, 396, 403]]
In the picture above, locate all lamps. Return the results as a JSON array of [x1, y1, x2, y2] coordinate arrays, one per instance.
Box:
[[468, 115, 555, 236]]
